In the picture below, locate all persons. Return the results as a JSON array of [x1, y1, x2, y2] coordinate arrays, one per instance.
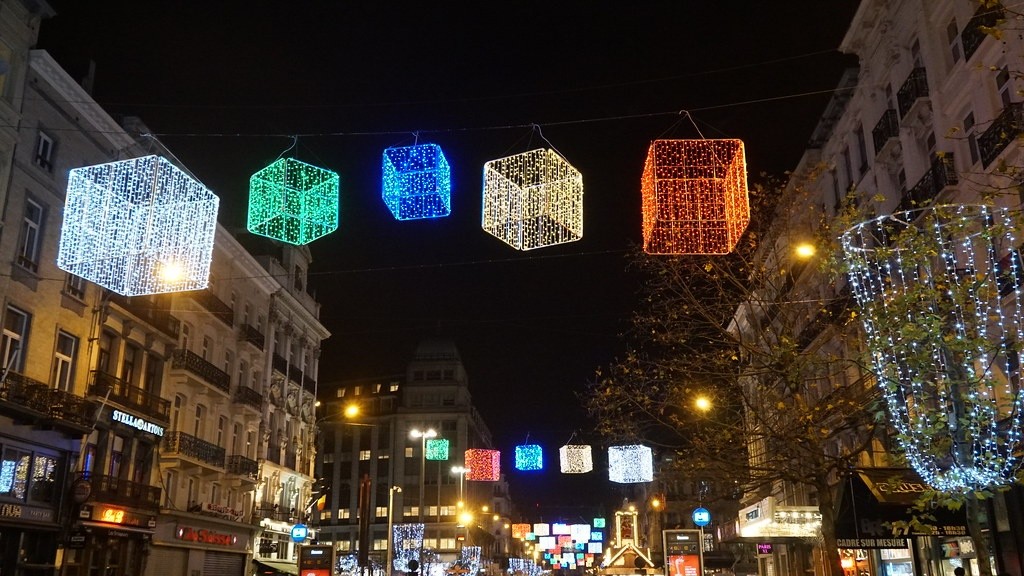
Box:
[[954, 567, 965, 576]]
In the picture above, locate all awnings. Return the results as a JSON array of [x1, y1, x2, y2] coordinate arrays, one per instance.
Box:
[[832, 467, 970, 548]]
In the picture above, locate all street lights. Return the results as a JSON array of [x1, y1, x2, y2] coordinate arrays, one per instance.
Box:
[[411, 429, 436, 576], [451, 467, 470, 562]]
